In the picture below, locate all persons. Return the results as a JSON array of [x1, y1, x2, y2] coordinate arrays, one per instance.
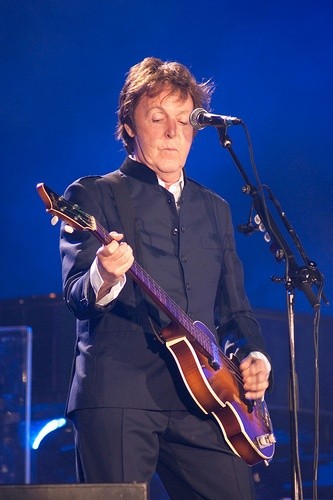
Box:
[[57, 55, 275, 499]]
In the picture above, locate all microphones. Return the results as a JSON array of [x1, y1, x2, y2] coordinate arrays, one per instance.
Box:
[[190, 107, 240, 130]]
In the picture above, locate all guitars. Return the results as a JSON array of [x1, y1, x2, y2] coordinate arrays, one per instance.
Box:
[[36, 183, 277, 467]]
